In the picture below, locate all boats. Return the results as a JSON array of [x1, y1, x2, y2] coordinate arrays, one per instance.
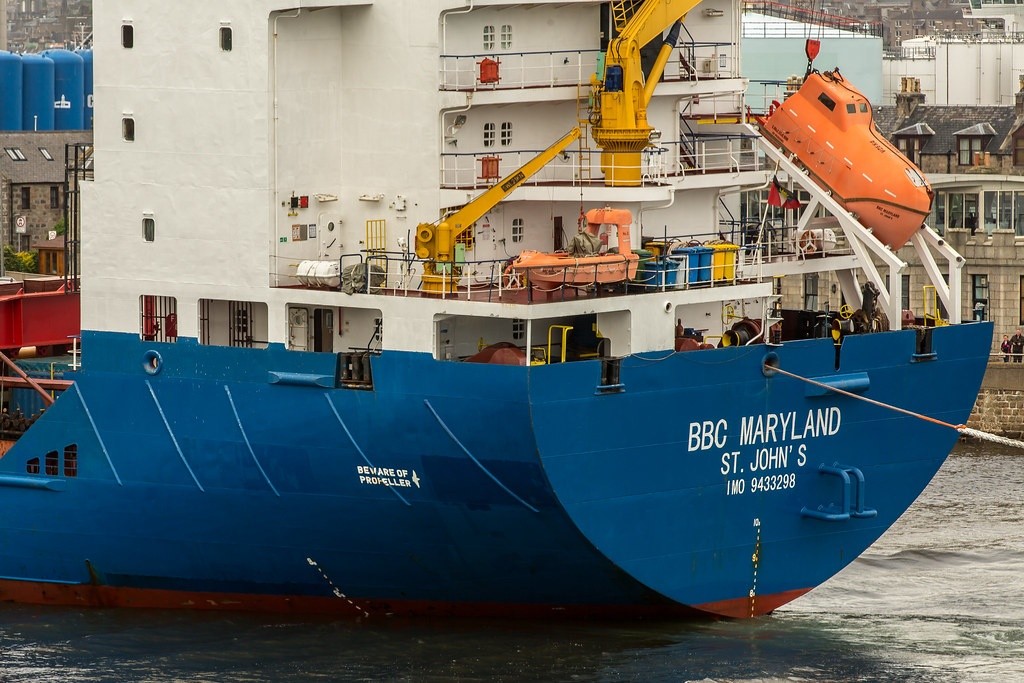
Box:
[[0, 2, 994, 617], [513, 208, 639, 289]]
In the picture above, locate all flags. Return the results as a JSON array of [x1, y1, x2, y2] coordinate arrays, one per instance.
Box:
[[769, 176, 802, 209]]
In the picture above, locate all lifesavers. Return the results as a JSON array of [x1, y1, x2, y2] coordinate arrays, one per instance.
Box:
[[800, 230, 817, 255], [503, 264, 527, 292]]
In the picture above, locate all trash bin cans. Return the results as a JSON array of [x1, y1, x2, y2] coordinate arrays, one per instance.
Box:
[[630, 241, 741, 293]]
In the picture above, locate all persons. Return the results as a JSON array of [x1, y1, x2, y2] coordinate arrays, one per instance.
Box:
[[1009, 329, 1024, 363], [1001, 335, 1012, 362]]
